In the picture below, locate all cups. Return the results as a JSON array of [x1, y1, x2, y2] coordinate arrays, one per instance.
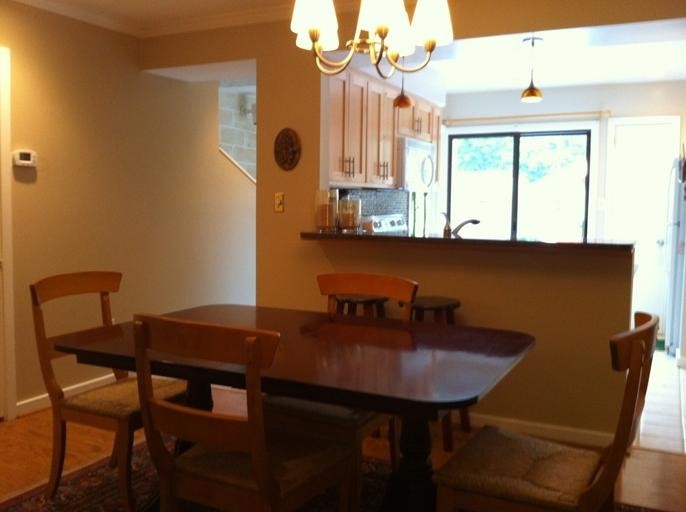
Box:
[[314, 189, 339, 234], [339, 199, 361, 233]]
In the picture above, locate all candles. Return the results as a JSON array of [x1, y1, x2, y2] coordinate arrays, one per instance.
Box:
[[317, 203, 334, 229], [364, 223, 372, 229], [342, 210, 354, 227]]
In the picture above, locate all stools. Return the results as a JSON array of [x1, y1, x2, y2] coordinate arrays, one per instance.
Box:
[[398, 295, 470, 453], [335, 291, 390, 428]]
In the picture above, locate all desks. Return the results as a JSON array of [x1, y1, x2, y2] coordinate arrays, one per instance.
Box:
[[55, 304, 538, 512]]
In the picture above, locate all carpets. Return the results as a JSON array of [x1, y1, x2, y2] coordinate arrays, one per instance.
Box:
[[0, 433, 437, 512]]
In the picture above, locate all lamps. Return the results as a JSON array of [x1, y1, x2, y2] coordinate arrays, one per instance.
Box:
[[518, 37, 546, 104], [393, 57, 413, 109], [291, 0, 455, 80]]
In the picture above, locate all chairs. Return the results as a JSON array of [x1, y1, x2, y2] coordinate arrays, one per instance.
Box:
[[134, 313, 358, 512], [30, 272, 188, 512], [261, 272, 419, 466], [432, 312, 659, 512]]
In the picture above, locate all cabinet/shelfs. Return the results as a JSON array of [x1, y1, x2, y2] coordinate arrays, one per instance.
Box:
[[398, 99, 432, 142], [321, 77, 367, 189], [366, 89, 398, 189], [433, 107, 439, 181]]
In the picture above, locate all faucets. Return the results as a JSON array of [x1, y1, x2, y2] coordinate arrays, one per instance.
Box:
[[452, 218, 482, 239]]
